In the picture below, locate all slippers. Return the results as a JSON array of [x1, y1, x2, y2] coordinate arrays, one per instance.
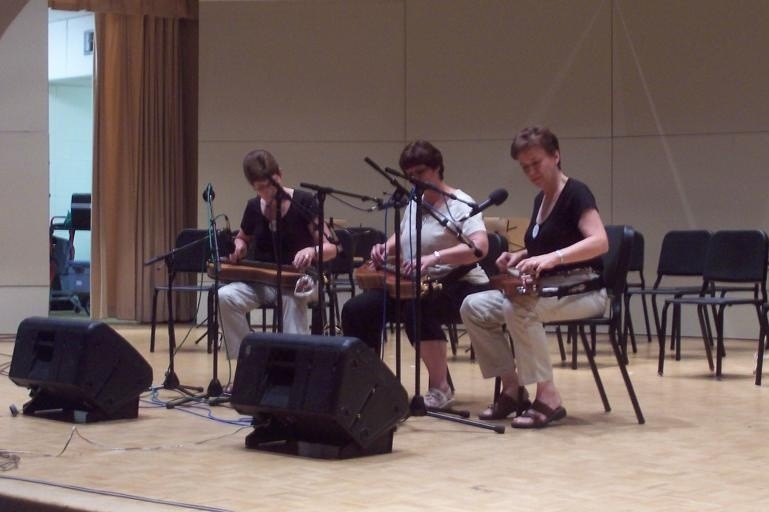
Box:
[[479, 392, 531, 420], [511, 399, 566, 427]]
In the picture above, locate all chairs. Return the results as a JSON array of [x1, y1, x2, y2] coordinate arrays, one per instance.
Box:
[[624, 229, 725, 369], [150, 225, 652, 371], [656, 226, 768, 386], [491, 227, 646, 425]]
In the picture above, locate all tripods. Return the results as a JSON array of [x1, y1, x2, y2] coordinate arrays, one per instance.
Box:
[[405, 196, 506, 434], [145, 258, 203, 397], [166, 205, 231, 409]]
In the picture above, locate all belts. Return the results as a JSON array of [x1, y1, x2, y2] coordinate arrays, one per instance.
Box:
[[542, 267, 597, 276]]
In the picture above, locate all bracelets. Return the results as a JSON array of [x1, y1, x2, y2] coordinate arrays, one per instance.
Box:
[[433, 249, 442, 265], [556, 249, 564, 266]]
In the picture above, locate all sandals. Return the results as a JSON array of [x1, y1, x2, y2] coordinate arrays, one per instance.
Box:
[[424, 386, 455, 409]]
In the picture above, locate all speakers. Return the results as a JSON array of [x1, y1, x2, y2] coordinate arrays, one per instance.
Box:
[[8, 316, 153, 424], [231, 331, 409, 461]]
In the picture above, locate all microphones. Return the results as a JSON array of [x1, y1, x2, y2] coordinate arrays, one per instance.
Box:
[[459, 187, 509, 223], [202, 183, 215, 203], [457, 233, 482, 258], [368, 198, 410, 213]]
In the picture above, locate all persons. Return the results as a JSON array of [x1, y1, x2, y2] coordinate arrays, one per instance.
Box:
[[215, 148, 337, 396], [341, 140, 490, 411], [458, 123, 610, 428]]
